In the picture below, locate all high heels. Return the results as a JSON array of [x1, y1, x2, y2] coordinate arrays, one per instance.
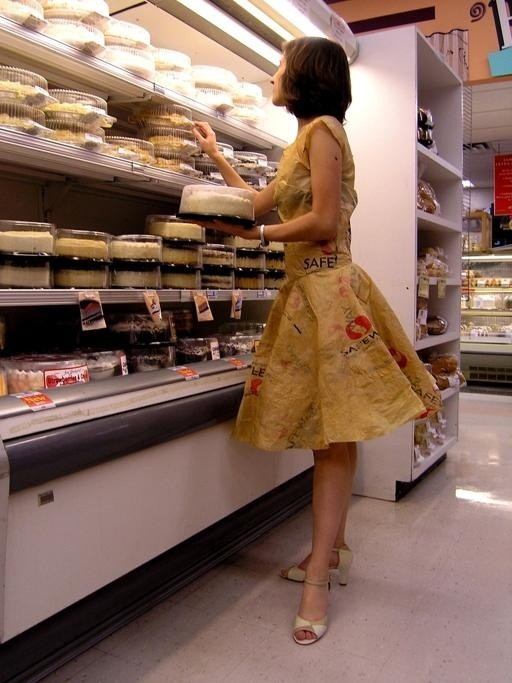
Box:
[[279, 544, 352, 586], [290, 572, 331, 645]]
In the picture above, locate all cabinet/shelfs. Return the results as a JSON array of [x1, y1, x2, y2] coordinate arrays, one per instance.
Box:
[[341, 27, 464, 502], [1, 0, 363, 683], [461, 253, 512, 390]]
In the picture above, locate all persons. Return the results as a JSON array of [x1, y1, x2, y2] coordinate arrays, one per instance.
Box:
[[191, 37, 442, 646]]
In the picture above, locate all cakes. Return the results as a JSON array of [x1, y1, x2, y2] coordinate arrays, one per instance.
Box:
[[1, 308, 267, 396], [1, 66, 279, 192], [0, 1, 265, 127], [176, 184, 256, 224], [0, 214, 287, 290]]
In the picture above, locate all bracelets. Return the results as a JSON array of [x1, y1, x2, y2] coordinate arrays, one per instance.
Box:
[[259, 224, 268, 249]]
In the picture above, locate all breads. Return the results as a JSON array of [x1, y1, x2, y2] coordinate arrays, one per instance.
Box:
[[415, 109, 466, 465]]
